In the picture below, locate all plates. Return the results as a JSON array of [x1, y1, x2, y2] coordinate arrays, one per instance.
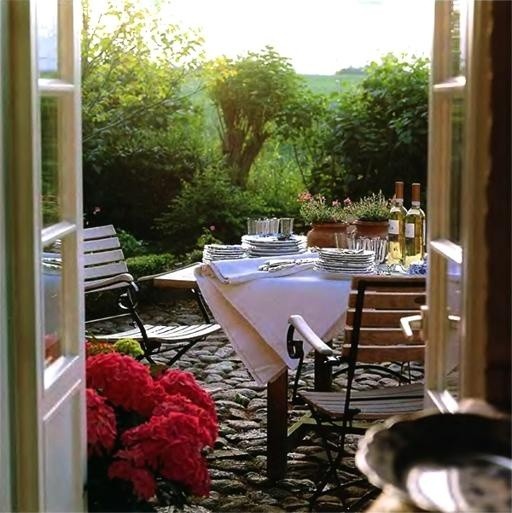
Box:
[[241, 235, 307, 258], [202, 245, 245, 260], [313, 248, 375, 275]]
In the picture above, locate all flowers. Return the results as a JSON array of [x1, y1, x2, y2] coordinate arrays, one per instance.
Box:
[[348, 189, 397, 221], [293, 192, 353, 225], [43, 334, 218, 501]]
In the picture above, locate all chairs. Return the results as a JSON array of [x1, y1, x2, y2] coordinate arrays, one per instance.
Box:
[[84, 222, 225, 366], [287, 272, 425, 510]]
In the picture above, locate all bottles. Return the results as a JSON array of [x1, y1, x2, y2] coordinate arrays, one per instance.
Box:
[[387, 182, 408, 264], [405, 183, 426, 262]]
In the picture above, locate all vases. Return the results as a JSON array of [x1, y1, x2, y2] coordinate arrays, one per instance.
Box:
[[351, 217, 390, 239], [306, 222, 349, 248]]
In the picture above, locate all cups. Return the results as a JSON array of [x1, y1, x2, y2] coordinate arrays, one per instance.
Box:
[[256, 221, 262, 237], [372, 240, 379, 258], [378, 240, 387, 263], [271, 219, 279, 237], [361, 239, 372, 250], [347, 232, 355, 249], [247, 217, 256, 235], [281, 218, 294, 238], [262, 220, 269, 237], [356, 239, 361, 250], [335, 232, 346, 250]]
